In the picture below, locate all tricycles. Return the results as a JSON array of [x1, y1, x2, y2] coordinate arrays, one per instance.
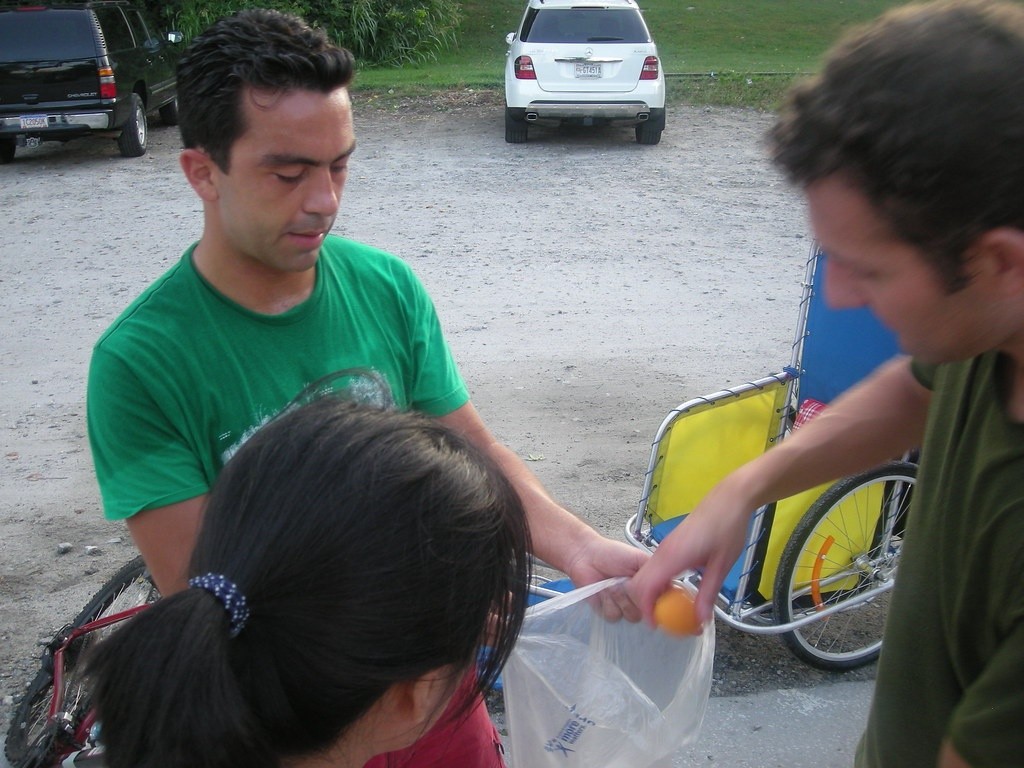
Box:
[[4, 236, 924, 768]]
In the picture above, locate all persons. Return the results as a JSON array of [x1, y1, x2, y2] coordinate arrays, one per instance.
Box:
[[87, 10, 652, 768], [624, 0, 1024, 768], [77, 366, 534, 768]]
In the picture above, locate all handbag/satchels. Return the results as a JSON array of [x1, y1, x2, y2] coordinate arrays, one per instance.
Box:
[[502, 571, 716, 768]]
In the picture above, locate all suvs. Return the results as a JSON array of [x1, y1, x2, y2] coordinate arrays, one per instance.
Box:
[[505, 0, 666, 144], [0, 0, 185, 162]]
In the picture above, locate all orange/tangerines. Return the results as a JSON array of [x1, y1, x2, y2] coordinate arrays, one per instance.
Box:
[[653, 588, 697, 636]]
